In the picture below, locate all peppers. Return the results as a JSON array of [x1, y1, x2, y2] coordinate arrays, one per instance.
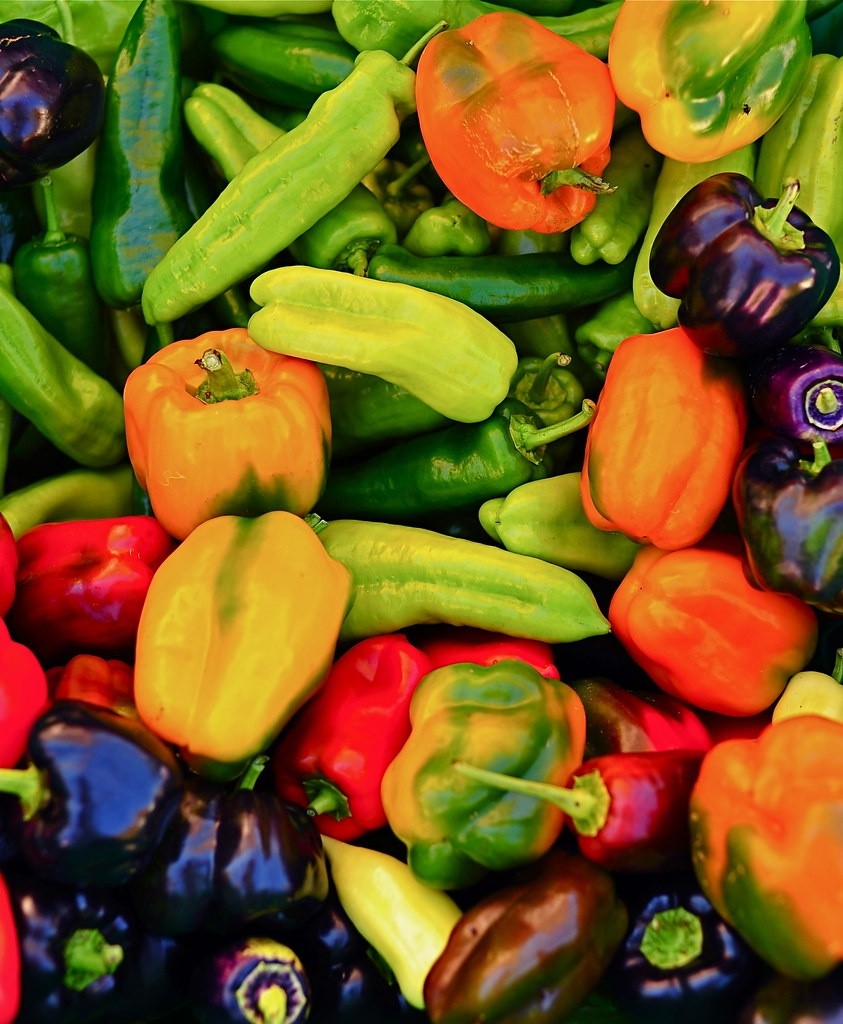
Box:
[[0, 0, 843, 1024]]
